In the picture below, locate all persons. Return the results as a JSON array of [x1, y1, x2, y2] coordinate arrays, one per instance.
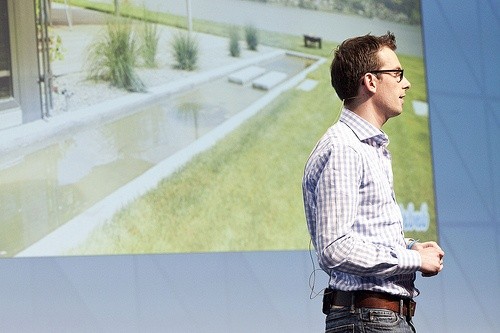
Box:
[[301, 29, 444, 333]]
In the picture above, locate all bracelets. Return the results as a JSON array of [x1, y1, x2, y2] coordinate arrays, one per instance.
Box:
[[408, 238, 421, 250]]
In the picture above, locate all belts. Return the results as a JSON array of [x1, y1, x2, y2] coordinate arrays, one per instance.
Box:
[[331, 291, 417, 317]]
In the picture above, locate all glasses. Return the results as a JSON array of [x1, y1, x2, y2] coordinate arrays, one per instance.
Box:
[[362, 68, 404, 85]]
